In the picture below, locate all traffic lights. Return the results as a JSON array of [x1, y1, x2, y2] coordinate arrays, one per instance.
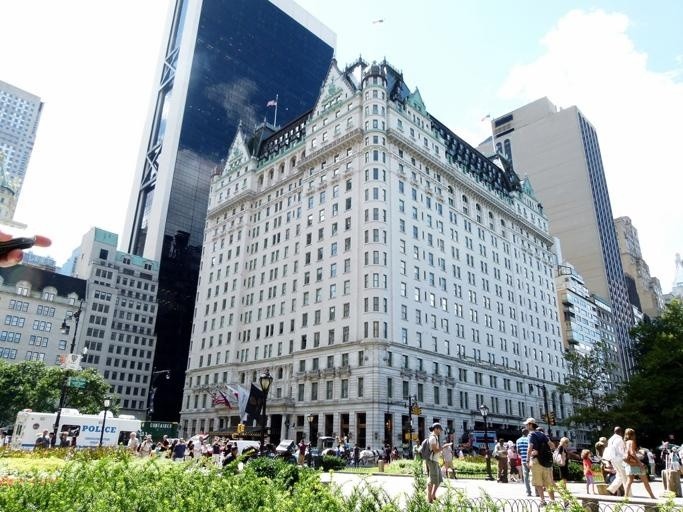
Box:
[[416, 406, 421, 415], [410, 405, 416, 414]]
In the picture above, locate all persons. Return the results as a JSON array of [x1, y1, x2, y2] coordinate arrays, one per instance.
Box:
[[663, 446, 683, 483], [0, 429, 9, 445], [426, 422, 453, 504], [60, 435, 70, 446], [582, 427, 656, 500], [336, 443, 361, 468], [413, 439, 423, 476], [493, 416, 570, 508], [296, 439, 306, 465], [128, 432, 238, 477], [1, 431, 7, 445], [381, 444, 398, 463], [35, 429, 50, 451], [443, 440, 456, 478], [71, 426, 80, 448], [49, 432, 56, 447]]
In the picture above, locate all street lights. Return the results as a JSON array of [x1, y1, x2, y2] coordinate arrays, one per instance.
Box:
[[146, 367, 172, 422], [255, 367, 273, 454], [306, 410, 314, 466], [386, 400, 407, 452], [96, 396, 112, 447], [596, 419, 603, 436], [478, 404, 494, 481], [51, 296, 86, 449], [528, 382, 553, 442]]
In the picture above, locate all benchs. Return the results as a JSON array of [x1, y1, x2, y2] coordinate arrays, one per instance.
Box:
[[574, 493, 661, 512]]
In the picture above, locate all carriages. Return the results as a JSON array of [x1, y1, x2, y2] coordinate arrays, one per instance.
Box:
[[315, 435, 386, 468]]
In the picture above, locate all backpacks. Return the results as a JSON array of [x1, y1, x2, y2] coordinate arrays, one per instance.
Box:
[[529, 432, 552, 468]]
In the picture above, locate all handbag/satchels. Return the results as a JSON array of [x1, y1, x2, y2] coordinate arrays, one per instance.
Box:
[[623, 450, 639, 466], [492, 451, 499, 459], [552, 445, 566, 466], [417, 437, 431, 460]]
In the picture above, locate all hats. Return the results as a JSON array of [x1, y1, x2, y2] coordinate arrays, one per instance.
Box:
[[522, 417, 536, 425], [433, 423, 443, 431]]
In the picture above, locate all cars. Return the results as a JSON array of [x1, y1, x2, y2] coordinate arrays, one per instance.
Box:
[[566, 448, 581, 460]]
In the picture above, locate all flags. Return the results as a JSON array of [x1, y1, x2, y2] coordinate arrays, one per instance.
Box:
[[204, 382, 263, 421], [266, 99, 278, 106]]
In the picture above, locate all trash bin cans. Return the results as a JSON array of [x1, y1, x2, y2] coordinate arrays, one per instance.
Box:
[[378, 460, 384, 472]]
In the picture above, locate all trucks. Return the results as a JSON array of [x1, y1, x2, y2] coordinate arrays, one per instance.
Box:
[[10, 407, 143, 451], [455, 430, 498, 458]]
